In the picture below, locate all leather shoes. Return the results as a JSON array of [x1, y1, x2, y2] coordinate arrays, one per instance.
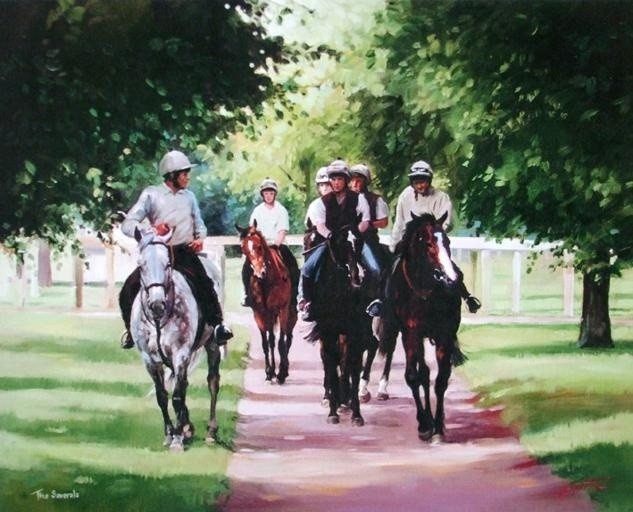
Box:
[[466, 296, 480, 311], [297, 298, 306, 312], [120, 333, 133, 350], [368, 302, 381, 317], [210, 325, 233, 346], [241, 295, 250, 305]]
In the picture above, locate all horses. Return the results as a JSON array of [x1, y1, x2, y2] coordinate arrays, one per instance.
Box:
[[128, 225, 224, 452], [384, 210, 469, 445], [303, 211, 393, 428], [232, 217, 302, 385]]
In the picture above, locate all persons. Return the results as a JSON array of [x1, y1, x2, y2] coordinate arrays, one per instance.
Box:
[[296, 160, 482, 322], [239, 179, 302, 307], [117, 148, 234, 349]]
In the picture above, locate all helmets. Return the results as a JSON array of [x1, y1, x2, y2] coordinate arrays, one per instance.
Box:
[[350, 164, 370, 185], [407, 167, 432, 179], [326, 160, 351, 183], [158, 151, 198, 177], [259, 180, 278, 196], [315, 166, 329, 185]]
